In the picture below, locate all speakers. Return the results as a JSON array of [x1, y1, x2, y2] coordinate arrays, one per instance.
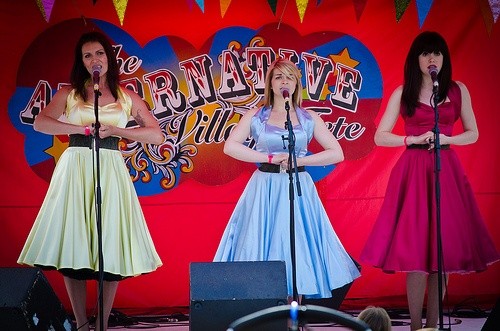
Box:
[[0, 267, 75, 331], [190, 261, 289, 331]]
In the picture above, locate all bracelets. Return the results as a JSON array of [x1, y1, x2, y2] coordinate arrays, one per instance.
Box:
[[84, 126, 90, 136], [268, 153, 273, 164], [404, 135, 409, 147]]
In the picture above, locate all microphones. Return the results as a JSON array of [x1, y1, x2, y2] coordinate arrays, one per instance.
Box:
[[429, 67, 439, 88], [280, 87, 290, 110], [92, 67, 102, 93]]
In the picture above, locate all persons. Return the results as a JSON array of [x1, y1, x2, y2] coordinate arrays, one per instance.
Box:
[[17, 31, 163, 331], [213, 58, 361, 304], [357, 307, 391, 331], [359, 31, 500, 330]]
